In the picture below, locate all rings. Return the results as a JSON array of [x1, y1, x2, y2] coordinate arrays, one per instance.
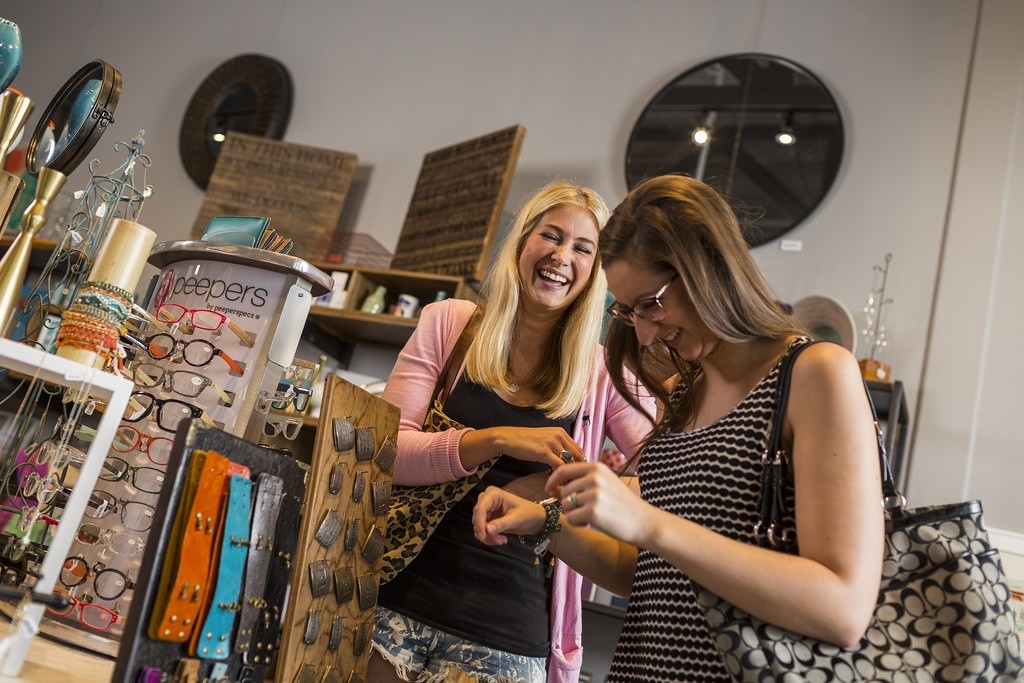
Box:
[[559, 449, 573, 463], [583, 456, 589, 463], [568, 492, 577, 507]]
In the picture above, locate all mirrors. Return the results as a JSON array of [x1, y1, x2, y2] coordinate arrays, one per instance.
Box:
[[623, 53, 846, 248], [179, 54, 292, 189]]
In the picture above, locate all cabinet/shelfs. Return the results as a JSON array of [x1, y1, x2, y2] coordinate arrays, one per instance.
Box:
[[259, 261, 488, 475], [582, 380, 911, 620], [0, 235, 106, 413]]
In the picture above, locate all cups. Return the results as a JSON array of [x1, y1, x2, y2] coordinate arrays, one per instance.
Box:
[[394, 294, 419, 319]]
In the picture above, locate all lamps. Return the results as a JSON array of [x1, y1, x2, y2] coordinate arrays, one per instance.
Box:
[[775, 112, 797, 145]]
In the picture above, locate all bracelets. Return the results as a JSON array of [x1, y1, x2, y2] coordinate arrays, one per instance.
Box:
[[519, 496, 564, 577], [544, 464, 569, 486]]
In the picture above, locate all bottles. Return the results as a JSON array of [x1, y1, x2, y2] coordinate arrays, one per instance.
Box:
[[360, 285, 386, 315]]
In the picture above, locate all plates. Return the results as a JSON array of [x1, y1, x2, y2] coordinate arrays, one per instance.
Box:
[[790, 295, 857, 354]]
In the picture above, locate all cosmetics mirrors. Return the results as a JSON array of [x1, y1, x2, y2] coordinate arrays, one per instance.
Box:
[[25, 59, 122, 180]]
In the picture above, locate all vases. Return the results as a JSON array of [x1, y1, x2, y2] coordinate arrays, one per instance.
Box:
[[360, 284, 387, 314]]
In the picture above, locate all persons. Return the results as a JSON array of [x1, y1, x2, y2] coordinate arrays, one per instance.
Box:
[[472, 174, 886, 683], [366, 178, 658, 683]]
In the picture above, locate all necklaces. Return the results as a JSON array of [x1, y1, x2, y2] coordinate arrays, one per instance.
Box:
[[511, 369, 530, 393]]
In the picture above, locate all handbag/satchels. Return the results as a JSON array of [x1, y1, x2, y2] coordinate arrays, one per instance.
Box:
[[689, 341, 1024, 683], [379, 304, 503, 587]]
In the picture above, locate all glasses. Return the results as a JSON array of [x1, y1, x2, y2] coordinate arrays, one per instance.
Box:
[[606, 272, 679, 326], [0, 388, 109, 589], [132, 363, 233, 407], [156, 304, 254, 348], [144, 333, 244, 376], [122, 391, 217, 434], [47, 588, 128, 632], [75, 523, 146, 557], [111, 424, 174, 465], [98, 456, 167, 494], [59, 556, 136, 601], [84, 490, 157, 532], [258, 367, 313, 458]]
